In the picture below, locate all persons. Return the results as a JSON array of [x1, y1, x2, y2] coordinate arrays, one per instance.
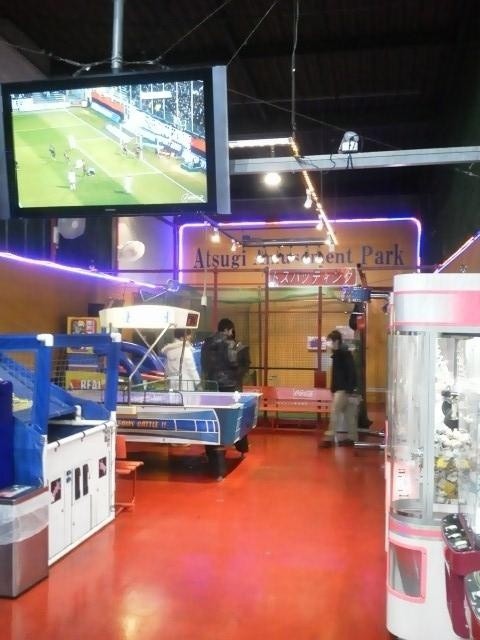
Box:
[[162, 328, 202, 392], [121, 142, 128, 157], [130, 80, 206, 131], [200, 318, 252, 453], [317, 330, 358, 448], [67, 168, 76, 191], [134, 145, 140, 158], [81, 159, 87, 175]]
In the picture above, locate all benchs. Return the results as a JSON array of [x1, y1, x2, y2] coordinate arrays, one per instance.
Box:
[[242, 385, 333, 432]]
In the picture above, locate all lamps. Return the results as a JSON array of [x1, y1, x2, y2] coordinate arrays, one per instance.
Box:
[[210, 145, 338, 265]]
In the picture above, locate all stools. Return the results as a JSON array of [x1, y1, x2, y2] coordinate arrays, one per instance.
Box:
[[116, 435, 145, 516]]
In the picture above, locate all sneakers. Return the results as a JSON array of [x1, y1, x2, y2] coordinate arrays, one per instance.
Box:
[[337, 439, 354, 447], [318, 440, 333, 449]]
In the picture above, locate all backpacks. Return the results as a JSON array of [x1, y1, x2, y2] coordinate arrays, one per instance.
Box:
[[223, 338, 251, 380]]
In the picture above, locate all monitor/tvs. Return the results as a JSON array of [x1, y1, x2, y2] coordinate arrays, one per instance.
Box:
[[1, 66, 232, 221]]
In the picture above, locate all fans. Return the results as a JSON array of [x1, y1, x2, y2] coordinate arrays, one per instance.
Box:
[[116, 241, 146, 263], [53, 218, 86, 249]]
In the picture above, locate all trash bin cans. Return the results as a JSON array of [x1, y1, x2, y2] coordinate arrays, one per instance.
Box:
[[0, 484, 49, 599]]
